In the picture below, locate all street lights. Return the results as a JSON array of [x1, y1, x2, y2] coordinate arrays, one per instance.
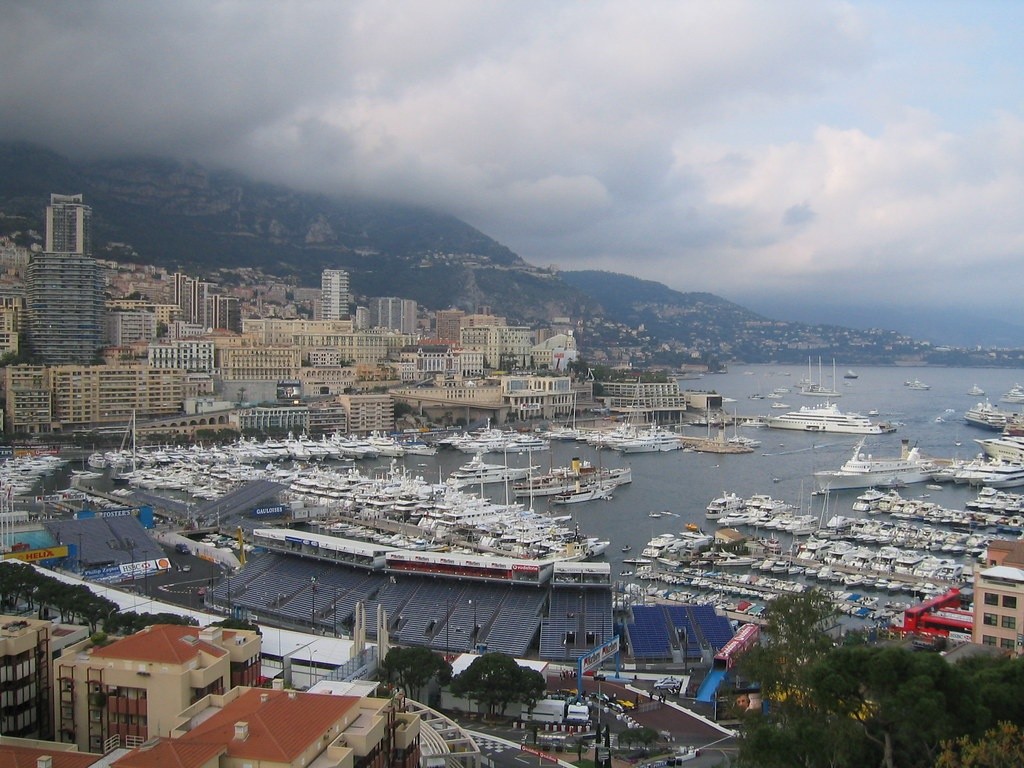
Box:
[[77, 533, 85, 574], [310, 575, 316, 634], [295, 642, 317, 687], [141, 550, 149, 596]]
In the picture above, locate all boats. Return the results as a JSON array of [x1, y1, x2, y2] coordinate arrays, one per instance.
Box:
[[0, 355, 1024, 631]]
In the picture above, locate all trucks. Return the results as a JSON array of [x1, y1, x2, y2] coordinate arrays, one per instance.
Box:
[[521, 698, 590, 725]]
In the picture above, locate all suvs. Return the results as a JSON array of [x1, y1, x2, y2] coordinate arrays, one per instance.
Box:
[[174, 543, 191, 556]]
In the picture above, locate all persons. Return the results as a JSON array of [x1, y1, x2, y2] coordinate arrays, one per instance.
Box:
[[559, 669, 684, 705]]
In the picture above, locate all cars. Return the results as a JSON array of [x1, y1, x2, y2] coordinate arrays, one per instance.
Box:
[[653, 676, 681, 691], [182, 563, 190, 572]]
